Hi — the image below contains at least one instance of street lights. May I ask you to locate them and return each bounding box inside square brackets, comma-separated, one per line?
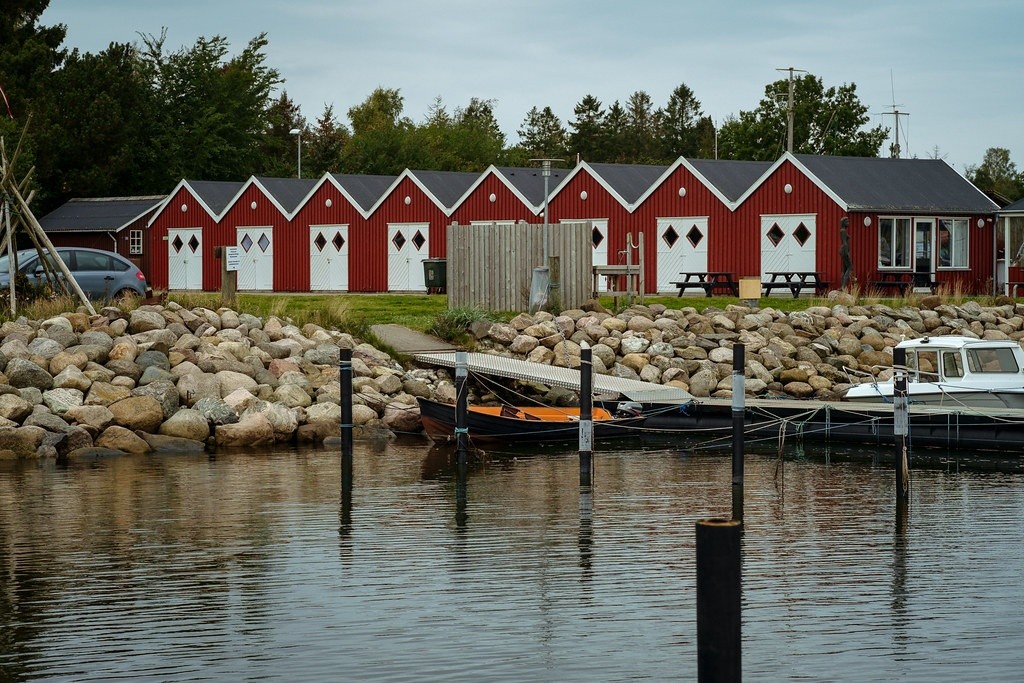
[288, 129, 302, 179]
[527, 157, 565, 268]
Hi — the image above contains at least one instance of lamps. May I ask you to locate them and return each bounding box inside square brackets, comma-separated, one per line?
[490, 193, 497, 202]
[404, 196, 411, 204]
[978, 219, 984, 228]
[580, 191, 588, 201]
[181, 204, 187, 212]
[785, 184, 793, 194]
[679, 188, 686, 197]
[864, 216, 872, 227]
[251, 201, 257, 209]
[326, 198, 332, 207]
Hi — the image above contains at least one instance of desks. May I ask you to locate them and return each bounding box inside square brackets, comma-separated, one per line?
[765, 272, 828, 299]
[875, 271, 938, 297]
[592, 264, 640, 298]
[677, 272, 739, 298]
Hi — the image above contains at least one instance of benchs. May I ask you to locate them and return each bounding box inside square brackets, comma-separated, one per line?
[670, 281, 947, 299]
[1006, 282, 1024, 299]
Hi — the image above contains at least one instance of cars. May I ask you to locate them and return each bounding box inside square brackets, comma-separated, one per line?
[0, 245, 148, 305]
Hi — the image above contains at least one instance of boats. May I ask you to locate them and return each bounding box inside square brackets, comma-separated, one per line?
[842, 334, 1024, 411]
[415, 393, 648, 448]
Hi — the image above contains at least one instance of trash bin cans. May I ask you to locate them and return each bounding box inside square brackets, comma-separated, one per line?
[422, 257, 448, 294]
